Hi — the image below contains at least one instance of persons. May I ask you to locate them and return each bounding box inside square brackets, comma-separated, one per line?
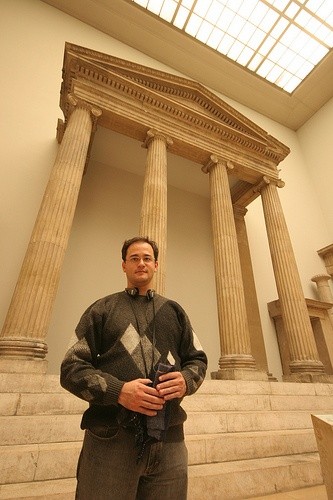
[59, 236, 208, 500]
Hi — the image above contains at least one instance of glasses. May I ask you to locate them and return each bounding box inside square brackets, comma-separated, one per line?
[124, 256, 154, 262]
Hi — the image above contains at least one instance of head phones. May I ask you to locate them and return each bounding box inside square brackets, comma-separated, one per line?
[125, 287, 155, 300]
